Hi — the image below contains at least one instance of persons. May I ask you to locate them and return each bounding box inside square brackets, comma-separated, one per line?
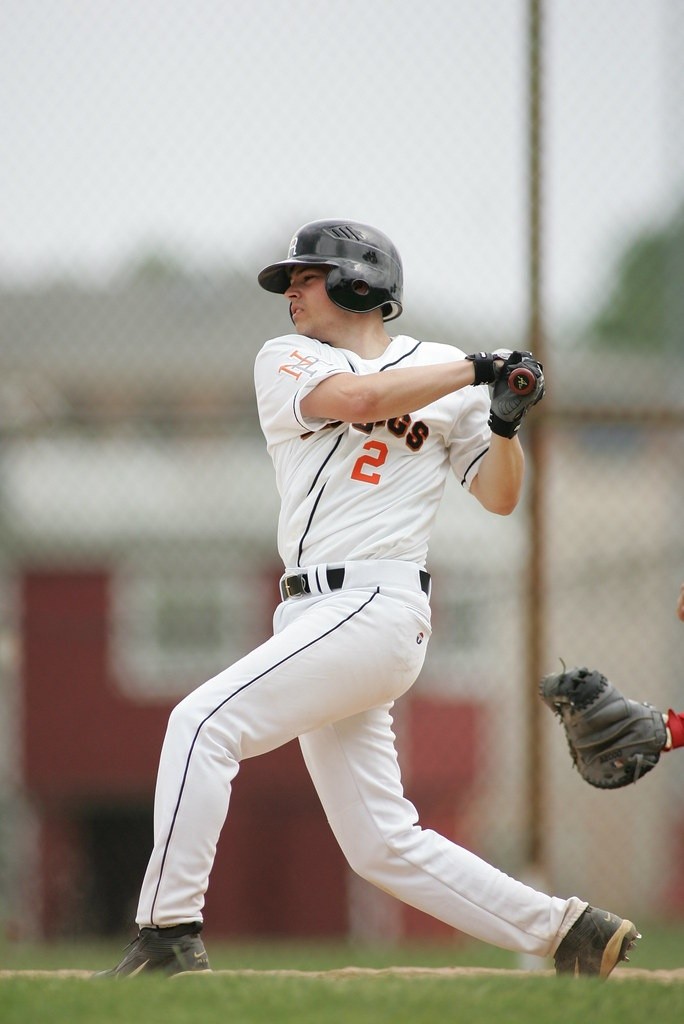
[101, 220, 642, 983]
[539, 580, 684, 789]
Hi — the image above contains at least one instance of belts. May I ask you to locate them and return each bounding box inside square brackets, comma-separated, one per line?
[280, 568, 431, 601]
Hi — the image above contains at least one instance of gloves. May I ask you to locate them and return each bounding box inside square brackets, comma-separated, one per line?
[464, 348, 543, 387]
[487, 350, 547, 440]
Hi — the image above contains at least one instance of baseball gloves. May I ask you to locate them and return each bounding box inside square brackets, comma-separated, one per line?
[537, 657, 668, 789]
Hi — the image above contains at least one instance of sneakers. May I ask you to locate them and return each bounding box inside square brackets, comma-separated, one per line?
[553, 904, 643, 984]
[89, 921, 213, 984]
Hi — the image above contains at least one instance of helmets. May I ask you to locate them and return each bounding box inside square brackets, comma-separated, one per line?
[257, 219, 404, 323]
